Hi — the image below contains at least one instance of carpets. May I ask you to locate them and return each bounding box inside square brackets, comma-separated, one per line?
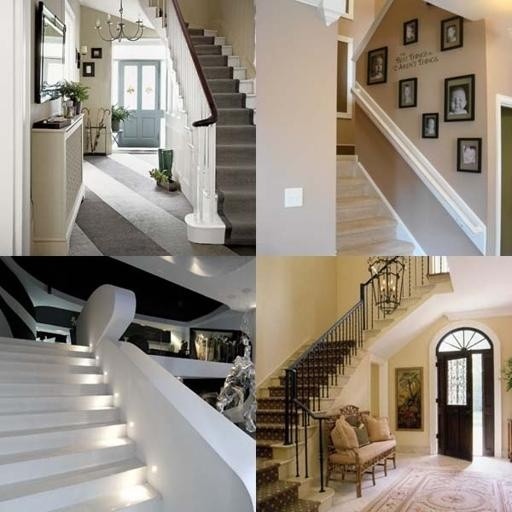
[356, 465, 512, 511]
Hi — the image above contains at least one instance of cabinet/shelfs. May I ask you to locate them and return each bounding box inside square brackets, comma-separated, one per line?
[31, 113, 85, 241]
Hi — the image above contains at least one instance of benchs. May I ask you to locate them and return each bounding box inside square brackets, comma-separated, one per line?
[322, 404, 398, 499]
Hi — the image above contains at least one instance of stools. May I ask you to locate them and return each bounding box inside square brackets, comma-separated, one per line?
[111, 129, 125, 148]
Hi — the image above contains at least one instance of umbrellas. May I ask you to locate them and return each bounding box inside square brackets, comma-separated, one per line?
[81, 105, 113, 153]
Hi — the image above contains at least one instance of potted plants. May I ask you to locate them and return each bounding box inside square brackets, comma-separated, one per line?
[111, 102, 130, 131]
[62, 79, 92, 114]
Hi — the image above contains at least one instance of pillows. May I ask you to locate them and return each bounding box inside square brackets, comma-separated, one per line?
[362, 413, 394, 442]
[328, 414, 359, 451]
[351, 422, 369, 449]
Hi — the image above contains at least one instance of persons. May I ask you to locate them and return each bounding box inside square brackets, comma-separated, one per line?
[461, 147, 475, 167]
[405, 23, 415, 41]
[445, 25, 457, 41]
[401, 84, 412, 101]
[370, 56, 383, 80]
[448, 86, 466, 116]
[422, 117, 436, 136]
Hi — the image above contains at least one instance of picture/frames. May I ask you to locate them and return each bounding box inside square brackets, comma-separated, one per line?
[402, 16, 420, 46]
[421, 112, 440, 139]
[456, 137, 482, 174]
[440, 14, 464, 52]
[444, 74, 477, 122]
[397, 76, 418, 110]
[367, 46, 388, 86]
[82, 62, 95, 77]
[91, 47, 103, 59]
[394, 366, 425, 432]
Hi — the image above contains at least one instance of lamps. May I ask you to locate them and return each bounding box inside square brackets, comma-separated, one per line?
[367, 256, 405, 316]
[75, 44, 88, 68]
[34, 1, 67, 105]
[93, 0, 145, 44]
